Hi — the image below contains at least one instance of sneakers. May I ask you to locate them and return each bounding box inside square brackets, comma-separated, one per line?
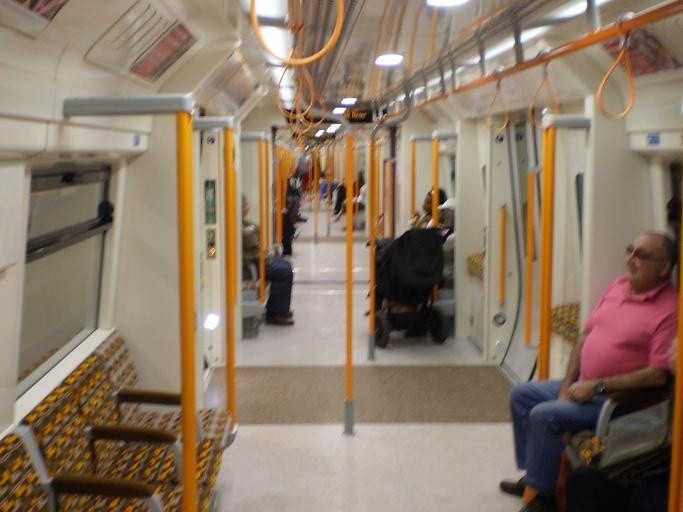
[264, 308, 295, 326]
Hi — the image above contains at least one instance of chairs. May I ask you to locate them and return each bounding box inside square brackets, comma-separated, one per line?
[23, 328, 235, 495]
[243, 248, 280, 338]
[0, 431, 166, 512]
[563, 375, 673, 512]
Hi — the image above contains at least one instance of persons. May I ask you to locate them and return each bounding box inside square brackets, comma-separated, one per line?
[498, 226, 681, 512]
[433, 202, 454, 290]
[240, 193, 298, 328]
[282, 161, 370, 259]
[366, 187, 447, 317]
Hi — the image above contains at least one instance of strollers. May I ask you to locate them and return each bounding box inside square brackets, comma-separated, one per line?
[364, 225, 455, 349]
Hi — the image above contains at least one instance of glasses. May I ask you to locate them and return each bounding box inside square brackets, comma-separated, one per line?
[625, 244, 671, 265]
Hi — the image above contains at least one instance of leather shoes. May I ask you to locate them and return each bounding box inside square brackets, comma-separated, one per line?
[499, 472, 531, 497]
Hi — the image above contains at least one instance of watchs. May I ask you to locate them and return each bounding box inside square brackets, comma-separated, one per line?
[594, 376, 611, 396]
